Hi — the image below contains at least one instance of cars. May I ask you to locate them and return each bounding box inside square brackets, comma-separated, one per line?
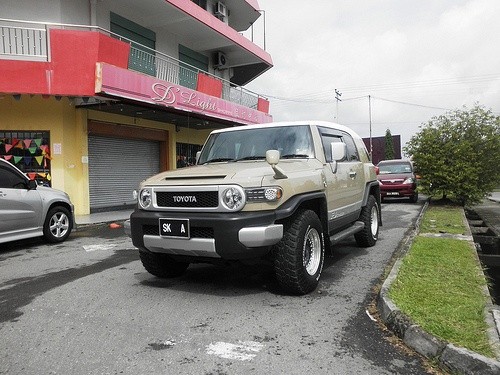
[375, 159, 421, 203]
[0, 159, 75, 245]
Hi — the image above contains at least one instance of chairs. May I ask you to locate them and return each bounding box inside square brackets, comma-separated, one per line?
[253, 141, 276, 155]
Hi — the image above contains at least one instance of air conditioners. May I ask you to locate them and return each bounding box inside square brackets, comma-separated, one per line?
[212, 51, 229, 71]
[213, 1, 227, 17]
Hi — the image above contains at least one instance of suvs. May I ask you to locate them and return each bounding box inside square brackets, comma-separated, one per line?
[129, 120, 382, 294]
